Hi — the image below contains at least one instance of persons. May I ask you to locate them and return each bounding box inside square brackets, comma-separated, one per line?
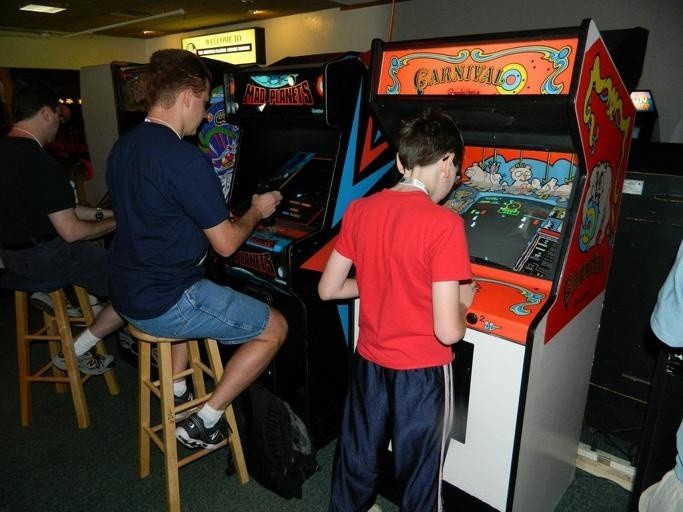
[101, 46, 293, 450]
[634, 240, 683, 512]
[1, 82, 128, 377]
[314, 111, 482, 511]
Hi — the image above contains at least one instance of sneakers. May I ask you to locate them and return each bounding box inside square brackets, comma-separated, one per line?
[28, 290, 105, 318]
[52, 348, 115, 375]
[173, 386, 195, 406]
[368, 504, 383, 512]
[174, 411, 229, 450]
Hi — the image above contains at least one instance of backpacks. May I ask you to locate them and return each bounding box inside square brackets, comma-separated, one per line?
[232, 379, 322, 501]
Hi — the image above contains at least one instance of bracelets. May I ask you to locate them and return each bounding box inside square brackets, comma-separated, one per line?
[95, 208, 103, 222]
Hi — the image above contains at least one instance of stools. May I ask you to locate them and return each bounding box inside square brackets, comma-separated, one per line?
[127, 322, 248, 512]
[2, 272, 119, 430]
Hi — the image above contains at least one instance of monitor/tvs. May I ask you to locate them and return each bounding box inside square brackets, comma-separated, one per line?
[271, 151, 335, 227]
[456, 196, 555, 272]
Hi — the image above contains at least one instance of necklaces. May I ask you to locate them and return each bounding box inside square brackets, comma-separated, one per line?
[145, 116, 181, 141]
[11, 126, 43, 150]
